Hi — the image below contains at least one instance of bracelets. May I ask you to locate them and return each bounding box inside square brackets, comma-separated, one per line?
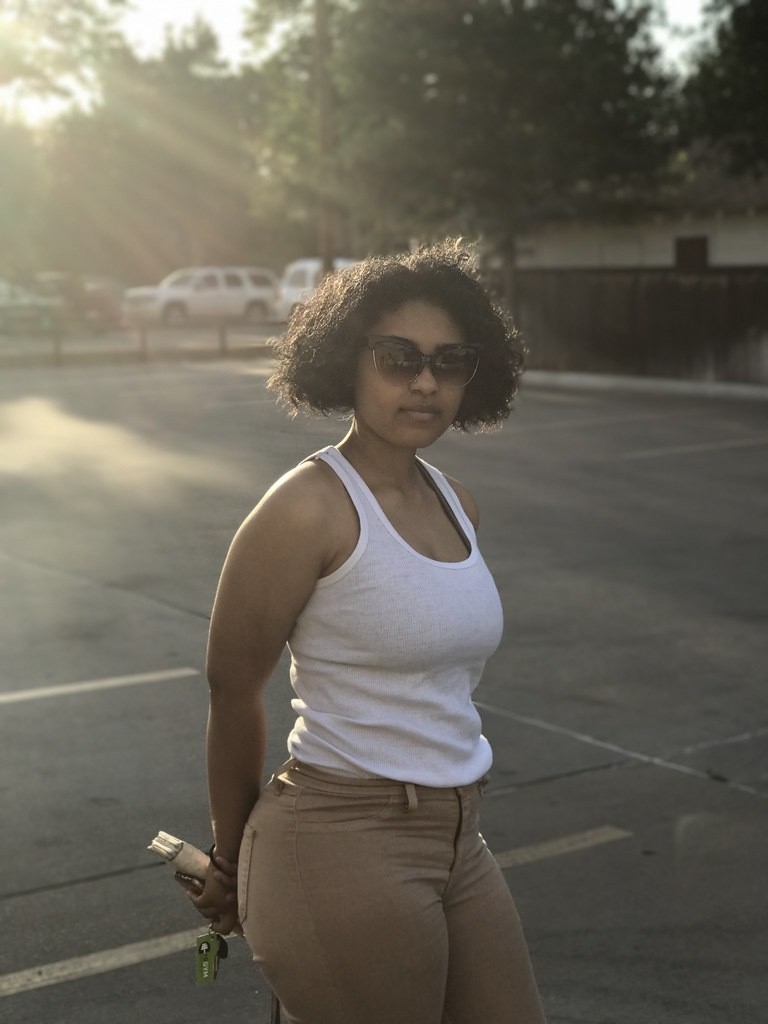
[210, 844, 238, 877]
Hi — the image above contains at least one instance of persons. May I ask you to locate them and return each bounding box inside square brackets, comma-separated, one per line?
[187, 234, 545, 1024]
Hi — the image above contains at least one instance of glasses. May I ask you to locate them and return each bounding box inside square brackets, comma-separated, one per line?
[362, 341, 484, 389]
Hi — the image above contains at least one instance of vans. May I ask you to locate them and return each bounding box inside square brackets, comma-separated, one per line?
[125, 265, 280, 328]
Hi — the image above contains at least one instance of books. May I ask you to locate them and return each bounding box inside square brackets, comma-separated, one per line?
[147, 831, 246, 939]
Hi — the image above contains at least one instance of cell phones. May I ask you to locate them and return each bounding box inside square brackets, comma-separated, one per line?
[173, 871, 205, 894]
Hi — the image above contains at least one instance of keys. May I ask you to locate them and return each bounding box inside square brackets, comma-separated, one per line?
[195, 921, 228, 985]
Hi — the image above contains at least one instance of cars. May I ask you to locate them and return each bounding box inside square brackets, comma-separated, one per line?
[266, 261, 366, 326]
